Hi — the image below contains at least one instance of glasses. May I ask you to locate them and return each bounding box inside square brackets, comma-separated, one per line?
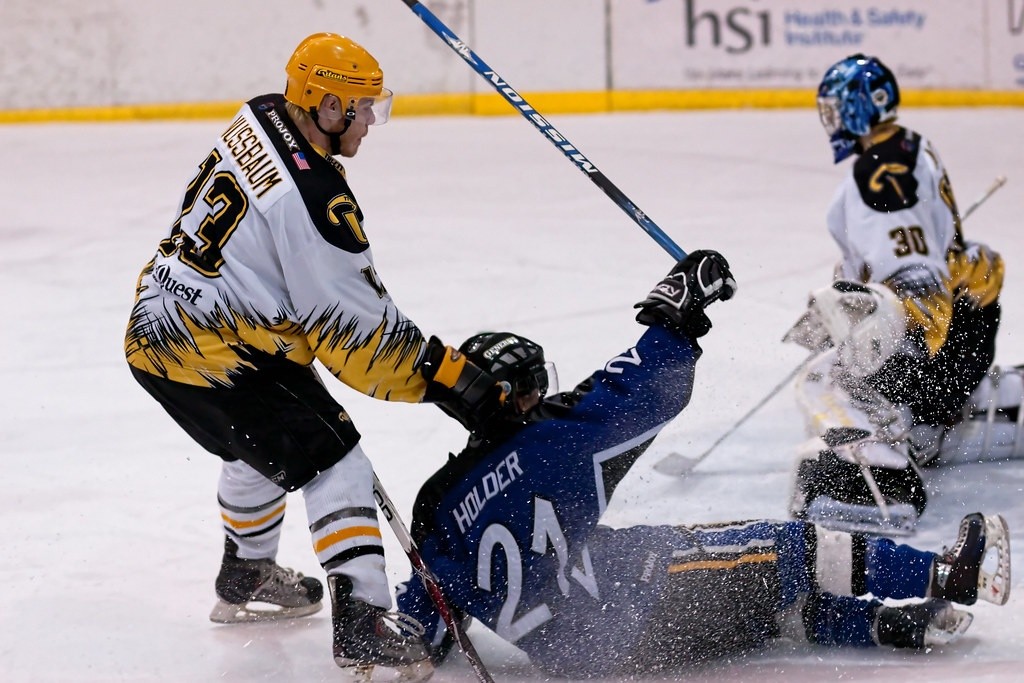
[816, 103, 841, 128]
[513, 368, 549, 396]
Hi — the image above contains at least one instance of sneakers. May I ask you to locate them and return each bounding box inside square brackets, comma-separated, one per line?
[210, 535, 325, 625]
[931, 512, 1011, 607]
[327, 574, 436, 683]
[878, 596, 974, 654]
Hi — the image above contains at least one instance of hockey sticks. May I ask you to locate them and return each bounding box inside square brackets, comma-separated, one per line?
[308, 362, 492, 683]
[402, 0, 736, 300]
[654, 175, 1005, 477]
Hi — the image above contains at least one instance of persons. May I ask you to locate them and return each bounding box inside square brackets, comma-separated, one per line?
[395, 249, 1010, 683]
[796, 53, 1024, 518]
[124, 32, 518, 683]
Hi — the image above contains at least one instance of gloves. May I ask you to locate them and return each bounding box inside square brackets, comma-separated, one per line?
[635, 249, 736, 340]
[420, 335, 508, 438]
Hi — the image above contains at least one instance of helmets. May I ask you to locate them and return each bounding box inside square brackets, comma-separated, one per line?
[816, 54, 900, 165]
[283, 32, 385, 117]
[456, 333, 546, 399]
